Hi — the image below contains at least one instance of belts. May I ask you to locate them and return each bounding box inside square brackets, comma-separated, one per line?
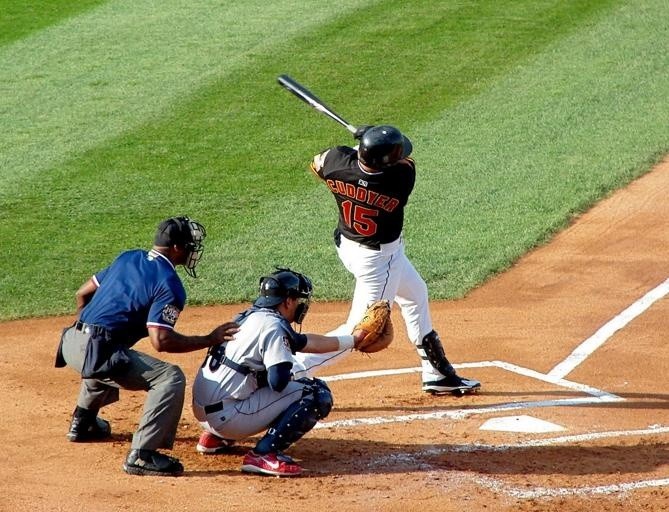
[69, 319, 130, 345]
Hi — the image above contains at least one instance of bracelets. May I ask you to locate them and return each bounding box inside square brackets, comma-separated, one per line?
[336, 334, 355, 351]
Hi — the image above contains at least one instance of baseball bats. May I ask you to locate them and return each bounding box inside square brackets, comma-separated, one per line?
[276, 74, 358, 133]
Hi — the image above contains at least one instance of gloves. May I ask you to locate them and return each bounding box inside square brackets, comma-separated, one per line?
[354, 125, 376, 140]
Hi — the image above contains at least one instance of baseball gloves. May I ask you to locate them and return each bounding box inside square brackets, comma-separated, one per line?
[351, 301, 394, 352]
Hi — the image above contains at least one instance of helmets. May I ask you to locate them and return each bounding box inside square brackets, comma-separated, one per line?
[356, 123, 415, 170]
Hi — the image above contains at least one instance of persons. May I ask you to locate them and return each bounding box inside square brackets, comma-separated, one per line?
[60, 217, 240, 477]
[285, 126, 484, 397]
[191, 266, 395, 478]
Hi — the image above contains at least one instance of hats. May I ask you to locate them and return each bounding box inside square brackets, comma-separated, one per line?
[252, 265, 308, 308]
[153, 217, 194, 248]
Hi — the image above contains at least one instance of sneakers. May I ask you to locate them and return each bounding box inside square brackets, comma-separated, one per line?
[65, 414, 114, 443]
[239, 449, 308, 476]
[421, 370, 483, 397]
[121, 447, 184, 477]
[196, 430, 238, 453]
